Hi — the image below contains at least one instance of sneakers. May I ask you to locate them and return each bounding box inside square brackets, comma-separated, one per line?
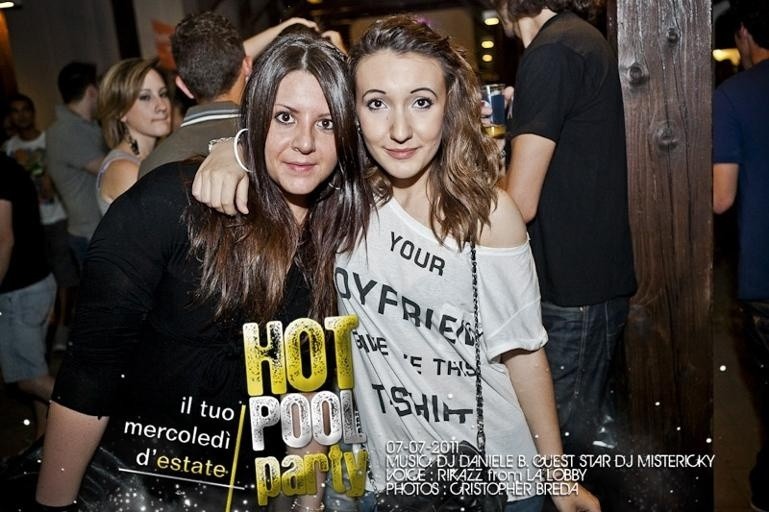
[751, 492, 769, 511]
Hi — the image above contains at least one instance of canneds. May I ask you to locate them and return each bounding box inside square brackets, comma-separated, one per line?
[480, 83, 508, 140]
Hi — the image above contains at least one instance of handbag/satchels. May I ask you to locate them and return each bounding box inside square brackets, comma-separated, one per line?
[375, 440, 508, 512]
[2, 433, 154, 511]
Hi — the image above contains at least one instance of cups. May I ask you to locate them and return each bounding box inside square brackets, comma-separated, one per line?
[480, 83, 509, 139]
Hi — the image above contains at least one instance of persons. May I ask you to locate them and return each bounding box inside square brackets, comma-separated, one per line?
[45, 62, 106, 272]
[189, 11, 605, 511]
[1, 148, 57, 469]
[94, 9, 360, 251]
[708, 11, 768, 511]
[32, 26, 370, 511]
[1, 92, 82, 357]
[487, 1, 630, 461]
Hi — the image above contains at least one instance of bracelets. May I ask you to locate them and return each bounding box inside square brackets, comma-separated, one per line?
[34, 499, 79, 511]
[206, 137, 236, 152]
[539, 455, 570, 476]
